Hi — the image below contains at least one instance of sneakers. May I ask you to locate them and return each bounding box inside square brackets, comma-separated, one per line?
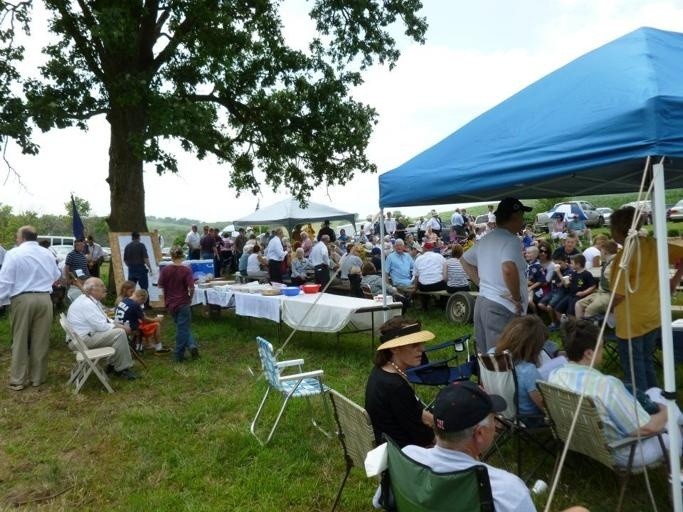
[6, 382, 41, 391]
[116, 344, 200, 381]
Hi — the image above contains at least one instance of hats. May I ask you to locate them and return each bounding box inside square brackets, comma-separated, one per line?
[170, 245, 183, 257]
[376, 322, 436, 351]
[497, 197, 532, 215]
[434, 380, 507, 433]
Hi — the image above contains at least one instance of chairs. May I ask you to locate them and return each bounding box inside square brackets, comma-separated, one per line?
[533, 374, 672, 509]
[475, 348, 538, 476]
[59, 312, 115, 395]
[250, 336, 334, 449]
[325, 389, 391, 510]
[380, 428, 501, 512]
[392, 331, 476, 416]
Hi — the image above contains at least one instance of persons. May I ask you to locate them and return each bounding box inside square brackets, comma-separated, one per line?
[459, 196, 533, 354]
[484, 314, 566, 422]
[188, 205, 623, 319]
[545, 317, 682, 475]
[1, 225, 200, 392]
[607, 206, 682, 392]
[370, 380, 589, 512]
[365, 314, 440, 455]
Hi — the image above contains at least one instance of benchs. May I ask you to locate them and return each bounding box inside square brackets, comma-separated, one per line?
[408, 255, 683, 344]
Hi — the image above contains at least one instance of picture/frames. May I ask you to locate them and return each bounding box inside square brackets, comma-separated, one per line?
[106, 230, 172, 312]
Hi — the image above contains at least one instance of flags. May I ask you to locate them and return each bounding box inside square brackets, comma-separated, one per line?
[69, 196, 85, 241]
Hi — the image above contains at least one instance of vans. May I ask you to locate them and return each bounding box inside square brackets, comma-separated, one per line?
[34, 235, 87, 260]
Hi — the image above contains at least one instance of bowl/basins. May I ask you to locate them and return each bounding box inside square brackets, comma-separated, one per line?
[282, 286, 300, 295]
[302, 283, 322, 294]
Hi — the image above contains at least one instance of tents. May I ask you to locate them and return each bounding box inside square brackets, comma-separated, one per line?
[379, 25, 682, 511]
[233, 195, 358, 242]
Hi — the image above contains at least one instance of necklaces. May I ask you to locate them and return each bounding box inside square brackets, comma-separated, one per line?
[386, 360, 409, 377]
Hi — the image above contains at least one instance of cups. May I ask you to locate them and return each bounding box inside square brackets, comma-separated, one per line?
[562, 276, 570, 286]
[532, 479, 547, 495]
[156, 315, 163, 323]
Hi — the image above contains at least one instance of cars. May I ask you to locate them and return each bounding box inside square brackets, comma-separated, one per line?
[160, 246, 188, 261]
[401, 197, 682, 241]
[101, 246, 111, 262]
